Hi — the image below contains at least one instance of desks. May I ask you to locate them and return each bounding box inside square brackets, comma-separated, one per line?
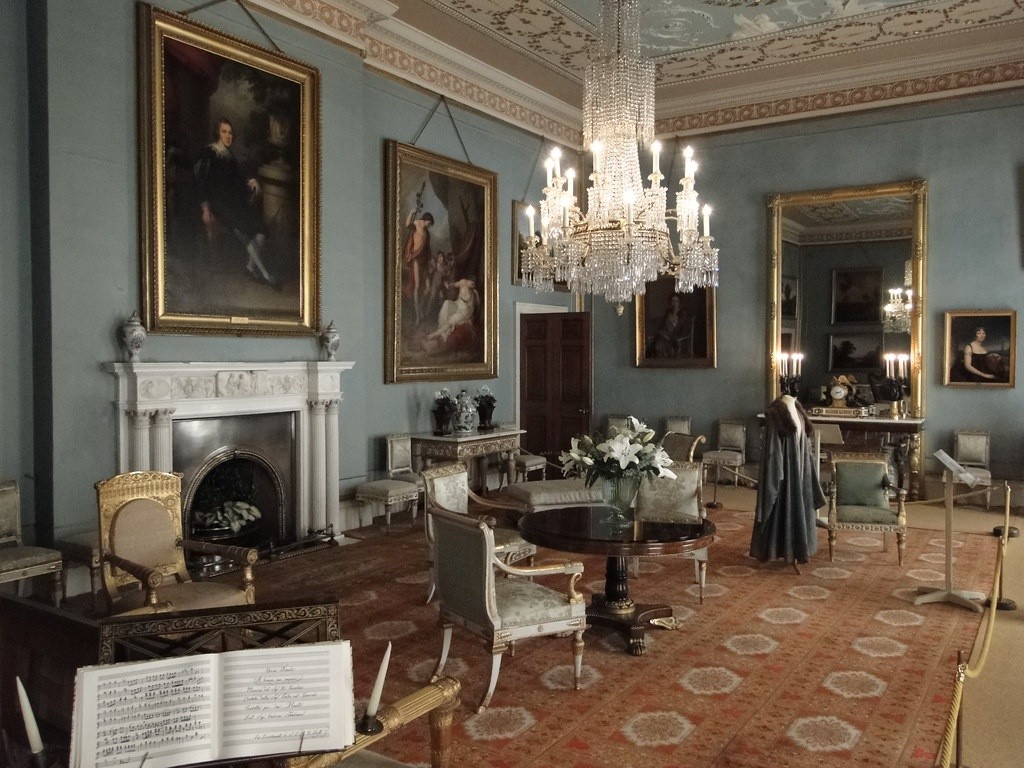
[759, 413, 925, 502]
[411, 427, 527, 495]
[518, 506, 716, 656]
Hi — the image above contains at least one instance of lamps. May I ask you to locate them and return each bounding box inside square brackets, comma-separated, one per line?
[884, 260, 912, 334]
[521, 0, 720, 316]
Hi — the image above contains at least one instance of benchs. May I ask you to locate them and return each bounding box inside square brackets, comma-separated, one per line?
[508, 477, 612, 506]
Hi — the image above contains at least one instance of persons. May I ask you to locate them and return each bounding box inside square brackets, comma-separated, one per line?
[750, 394, 826, 563]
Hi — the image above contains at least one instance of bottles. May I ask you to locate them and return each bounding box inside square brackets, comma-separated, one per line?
[319, 319, 342, 361]
[119, 309, 147, 364]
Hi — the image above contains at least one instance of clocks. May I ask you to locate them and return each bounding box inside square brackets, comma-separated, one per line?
[831, 384, 848, 407]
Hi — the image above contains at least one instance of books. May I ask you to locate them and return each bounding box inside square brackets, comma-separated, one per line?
[69, 641, 355, 768]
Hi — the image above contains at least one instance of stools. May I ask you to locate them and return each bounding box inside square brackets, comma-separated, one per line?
[356, 479, 418, 536]
[499, 455, 547, 492]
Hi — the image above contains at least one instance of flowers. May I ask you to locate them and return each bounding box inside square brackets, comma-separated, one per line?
[434, 386, 457, 409]
[558, 415, 678, 488]
[474, 385, 497, 406]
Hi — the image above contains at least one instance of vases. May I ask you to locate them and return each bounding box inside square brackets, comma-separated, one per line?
[598, 469, 641, 528]
[432, 410, 455, 436]
[477, 406, 497, 429]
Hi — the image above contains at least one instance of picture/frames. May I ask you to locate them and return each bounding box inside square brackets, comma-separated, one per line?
[136, 0, 322, 339]
[942, 309, 1017, 388]
[782, 275, 799, 320]
[511, 198, 572, 292]
[780, 328, 797, 375]
[828, 331, 885, 373]
[634, 271, 718, 371]
[831, 265, 885, 325]
[384, 139, 500, 385]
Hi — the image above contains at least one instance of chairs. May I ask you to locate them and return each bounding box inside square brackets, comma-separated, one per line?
[0, 480, 65, 607]
[418, 463, 537, 604]
[427, 508, 586, 715]
[942, 427, 992, 510]
[702, 419, 746, 488]
[94, 471, 260, 647]
[659, 431, 707, 462]
[664, 417, 691, 434]
[634, 461, 707, 584]
[385, 432, 428, 511]
[828, 452, 908, 565]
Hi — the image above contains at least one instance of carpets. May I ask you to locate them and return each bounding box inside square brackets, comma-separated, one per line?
[1, 484, 1002, 768]
[0, 591, 218, 768]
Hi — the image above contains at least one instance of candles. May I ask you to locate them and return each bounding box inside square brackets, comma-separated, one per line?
[15, 676, 43, 754]
[791, 353, 797, 377]
[766, 178, 928, 422]
[883, 354, 909, 381]
[367, 640, 392, 715]
[781, 353, 803, 376]
